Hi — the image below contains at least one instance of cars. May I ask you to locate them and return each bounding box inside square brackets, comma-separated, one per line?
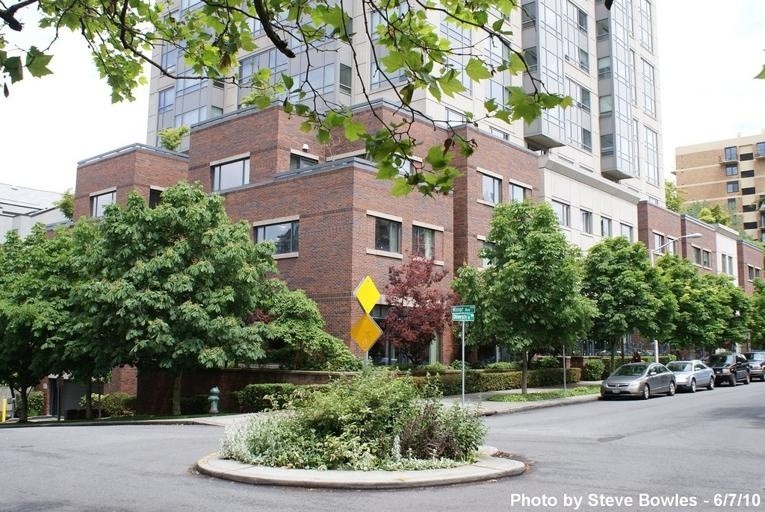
[588, 343, 669, 357]
[665, 359, 716, 393]
[598, 361, 678, 401]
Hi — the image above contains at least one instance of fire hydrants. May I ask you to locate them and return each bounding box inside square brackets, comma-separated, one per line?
[207, 386, 222, 414]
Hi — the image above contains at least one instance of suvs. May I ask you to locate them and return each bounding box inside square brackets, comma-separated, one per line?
[743, 350, 765, 383]
[705, 351, 753, 387]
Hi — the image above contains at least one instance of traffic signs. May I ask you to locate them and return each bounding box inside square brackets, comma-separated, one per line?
[452, 312, 475, 322]
[450, 304, 476, 314]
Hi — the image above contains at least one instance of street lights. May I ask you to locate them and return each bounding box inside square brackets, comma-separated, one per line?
[647, 231, 708, 369]
